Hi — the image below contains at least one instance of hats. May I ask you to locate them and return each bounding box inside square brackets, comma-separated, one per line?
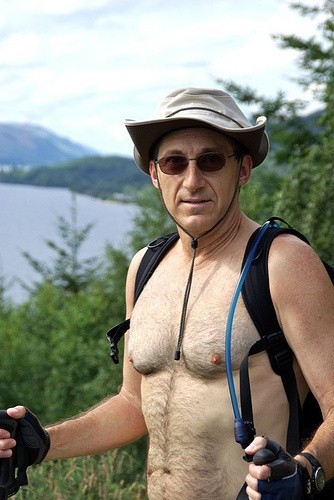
[123, 87, 269, 176]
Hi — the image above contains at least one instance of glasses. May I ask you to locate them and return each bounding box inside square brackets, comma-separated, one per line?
[155, 150, 240, 175]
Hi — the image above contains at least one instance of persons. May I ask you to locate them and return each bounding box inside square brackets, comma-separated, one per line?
[0, 90, 334, 499]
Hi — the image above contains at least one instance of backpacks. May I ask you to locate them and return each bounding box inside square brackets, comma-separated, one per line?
[108, 216, 334, 499]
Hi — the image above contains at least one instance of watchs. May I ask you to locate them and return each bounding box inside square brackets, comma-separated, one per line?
[299, 451, 327, 498]
